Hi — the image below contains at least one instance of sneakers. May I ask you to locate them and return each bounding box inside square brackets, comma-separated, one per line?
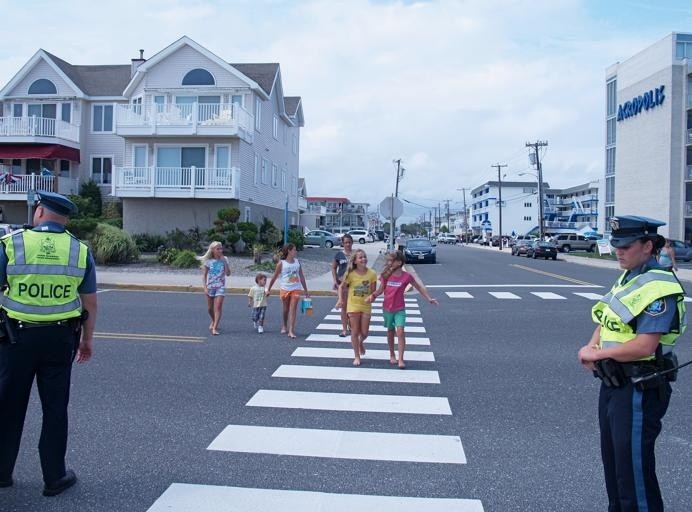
[339, 330, 348, 337]
[258, 326, 263, 333]
[348, 326, 351, 335]
[254, 321, 258, 328]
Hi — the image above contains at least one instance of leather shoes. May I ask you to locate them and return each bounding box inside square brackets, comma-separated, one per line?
[0, 480, 13, 488]
[43, 471, 76, 497]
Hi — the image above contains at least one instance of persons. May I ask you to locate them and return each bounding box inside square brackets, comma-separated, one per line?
[194, 241, 231, 335]
[0, 190, 97, 497]
[335, 249, 377, 365]
[656, 239, 678, 271]
[578, 213, 692, 512]
[332, 234, 357, 337]
[248, 273, 269, 333]
[265, 243, 308, 339]
[364, 250, 439, 368]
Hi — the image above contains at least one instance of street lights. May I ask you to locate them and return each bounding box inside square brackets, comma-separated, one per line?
[456, 186, 470, 244]
[518, 171, 545, 241]
[499, 171, 508, 239]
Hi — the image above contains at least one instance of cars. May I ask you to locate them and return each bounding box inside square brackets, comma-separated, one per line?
[437, 230, 457, 246]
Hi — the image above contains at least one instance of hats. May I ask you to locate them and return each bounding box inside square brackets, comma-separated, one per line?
[35, 190, 78, 217]
[605, 215, 666, 248]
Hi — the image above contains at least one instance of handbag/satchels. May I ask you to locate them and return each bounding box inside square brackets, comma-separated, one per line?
[302, 295, 312, 314]
